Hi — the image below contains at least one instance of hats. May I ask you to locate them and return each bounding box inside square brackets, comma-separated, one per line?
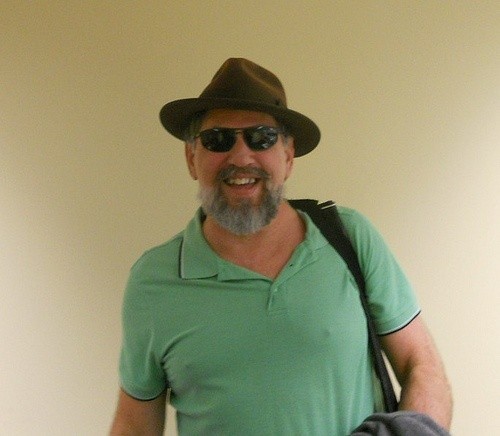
[160, 57, 321, 158]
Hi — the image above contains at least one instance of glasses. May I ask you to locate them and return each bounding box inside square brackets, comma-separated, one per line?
[194, 124, 288, 153]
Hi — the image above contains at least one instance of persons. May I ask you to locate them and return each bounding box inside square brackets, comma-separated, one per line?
[110, 57, 454, 436]
